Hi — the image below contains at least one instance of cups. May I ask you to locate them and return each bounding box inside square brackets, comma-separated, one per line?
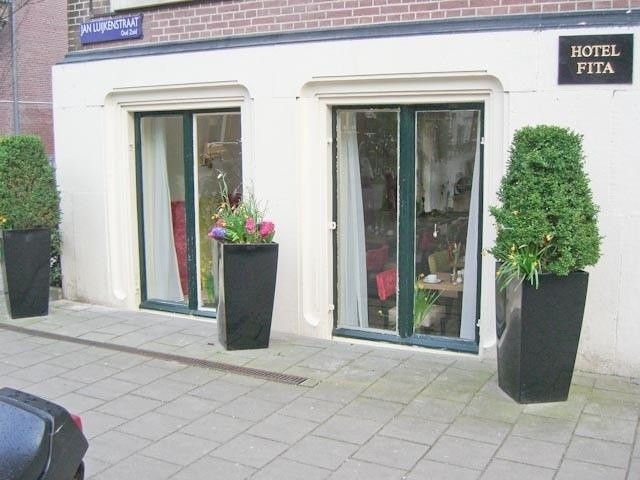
[425, 275, 438, 282]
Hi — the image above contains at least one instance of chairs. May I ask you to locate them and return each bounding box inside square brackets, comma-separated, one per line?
[364, 212, 466, 340]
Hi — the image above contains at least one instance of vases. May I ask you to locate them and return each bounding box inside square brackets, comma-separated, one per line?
[213, 241, 279, 352]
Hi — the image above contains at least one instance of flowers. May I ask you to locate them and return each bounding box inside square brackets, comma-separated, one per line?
[209, 169, 276, 245]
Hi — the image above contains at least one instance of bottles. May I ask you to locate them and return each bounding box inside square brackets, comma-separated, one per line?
[455, 270, 463, 286]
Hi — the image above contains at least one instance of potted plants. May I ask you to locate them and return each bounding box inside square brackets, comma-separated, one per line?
[486, 124, 605, 404]
[0, 134, 61, 319]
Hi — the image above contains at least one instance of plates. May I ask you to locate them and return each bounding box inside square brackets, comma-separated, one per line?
[423, 279, 441, 284]
